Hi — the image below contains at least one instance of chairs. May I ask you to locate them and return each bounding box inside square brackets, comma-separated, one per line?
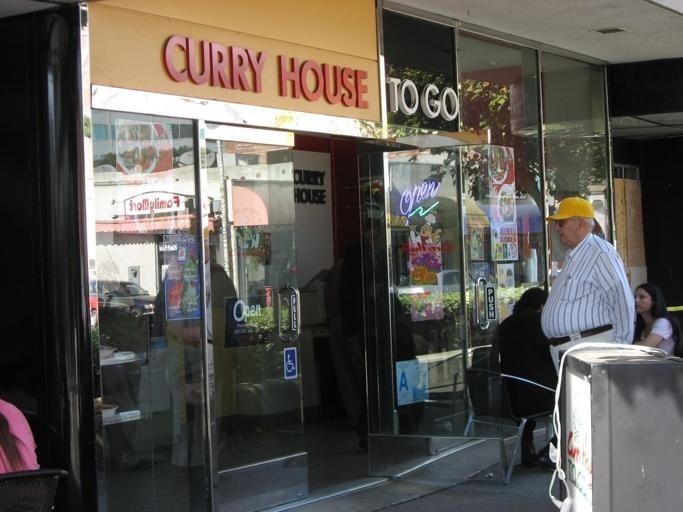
[0, 469, 70, 512]
[456, 362, 558, 486]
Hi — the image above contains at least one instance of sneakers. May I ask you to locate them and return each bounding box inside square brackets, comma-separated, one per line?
[520, 444, 539, 468]
[536, 442, 556, 472]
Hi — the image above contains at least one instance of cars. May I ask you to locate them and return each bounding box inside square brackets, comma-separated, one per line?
[394, 266, 489, 295]
[90, 276, 158, 336]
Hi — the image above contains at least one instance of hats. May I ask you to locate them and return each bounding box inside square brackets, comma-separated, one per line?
[544, 196, 596, 224]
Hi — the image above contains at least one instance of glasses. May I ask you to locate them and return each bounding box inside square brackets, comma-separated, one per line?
[553, 220, 578, 227]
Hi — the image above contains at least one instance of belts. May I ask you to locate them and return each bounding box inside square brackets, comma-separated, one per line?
[545, 322, 614, 349]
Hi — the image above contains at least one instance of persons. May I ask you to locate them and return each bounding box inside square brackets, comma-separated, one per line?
[633, 283, 683, 358]
[0, 397, 54, 512]
[541, 197, 634, 374]
[492, 288, 559, 471]
[152, 236, 246, 482]
[98, 267, 157, 472]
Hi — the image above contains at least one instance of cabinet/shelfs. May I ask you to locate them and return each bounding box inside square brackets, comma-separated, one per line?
[98, 306, 156, 429]
